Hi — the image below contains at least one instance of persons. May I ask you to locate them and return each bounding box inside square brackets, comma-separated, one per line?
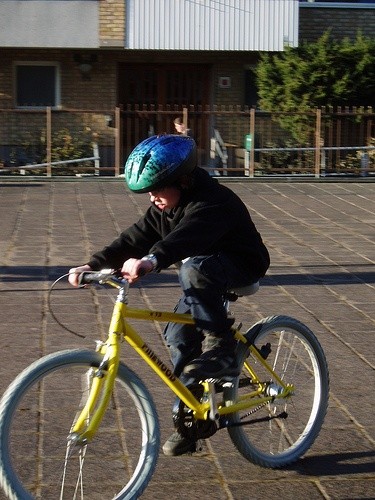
[174, 117, 192, 135]
[69, 134, 269, 456]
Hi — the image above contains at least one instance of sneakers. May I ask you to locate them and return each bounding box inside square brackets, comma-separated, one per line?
[161, 427, 195, 457]
[183, 328, 240, 375]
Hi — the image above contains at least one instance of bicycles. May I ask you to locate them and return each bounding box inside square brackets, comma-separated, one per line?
[0, 265, 330, 500]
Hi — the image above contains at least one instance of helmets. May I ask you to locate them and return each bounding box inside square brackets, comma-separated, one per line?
[124, 133, 197, 194]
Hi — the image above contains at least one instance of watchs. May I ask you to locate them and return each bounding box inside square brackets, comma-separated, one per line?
[142, 254, 158, 271]
